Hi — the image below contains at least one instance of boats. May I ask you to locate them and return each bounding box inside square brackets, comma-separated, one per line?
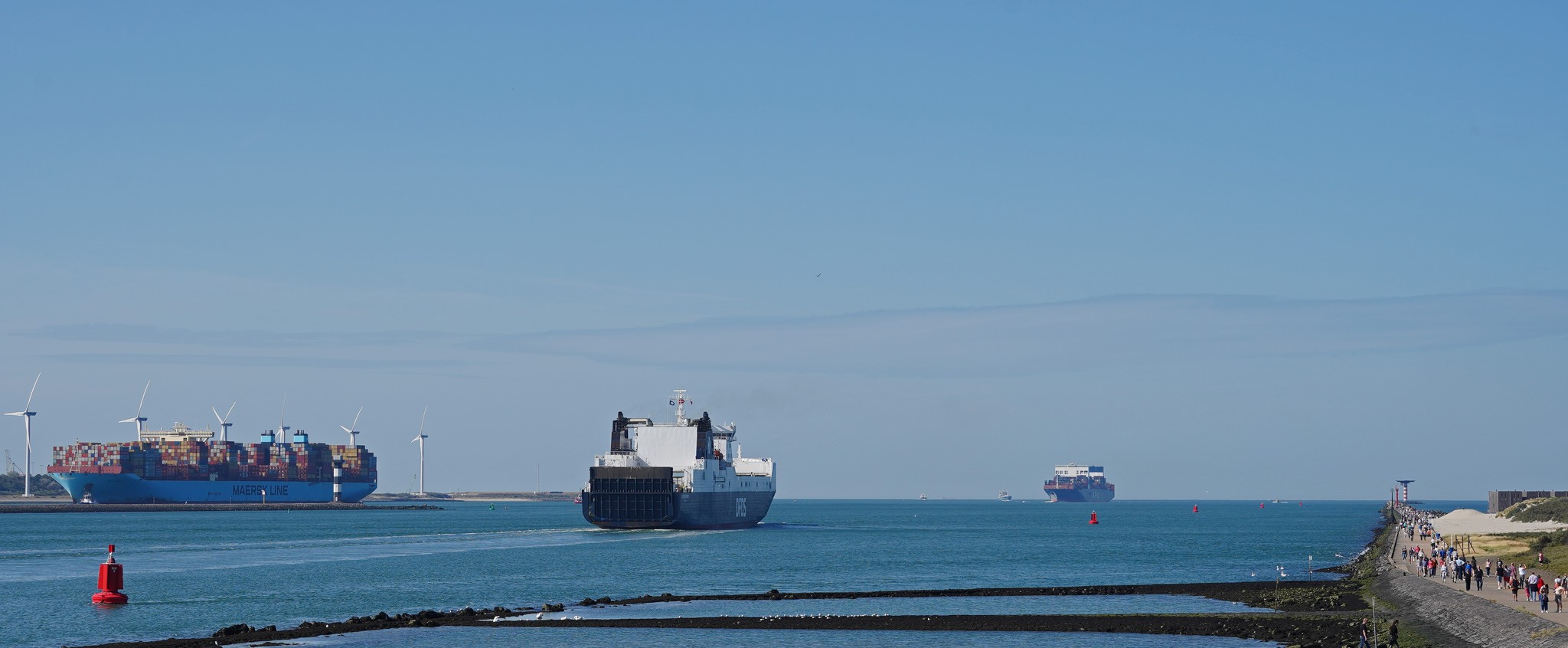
[1280, 501, 1289, 503]
[1044, 460, 1115, 502]
[920, 493, 927, 500]
[80, 491, 92, 504]
[1276, 498, 1280, 502]
[574, 487, 582, 503]
[582, 386, 777, 530]
[998, 488, 1012, 501]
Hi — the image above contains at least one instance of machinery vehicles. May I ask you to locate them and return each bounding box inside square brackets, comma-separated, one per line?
[406, 474, 416, 494]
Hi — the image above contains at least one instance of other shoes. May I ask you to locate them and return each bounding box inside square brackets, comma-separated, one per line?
[1403, 559, 1406, 562]
[1522, 588, 1524, 589]
[1546, 611, 1549, 613]
[1442, 581, 1445, 583]
[1566, 594, 1568, 598]
[1417, 574, 1421, 577]
[1477, 587, 1482, 591]
[1452, 580, 1456, 583]
[1432, 575, 1436, 577]
[1533, 600, 1536, 602]
[1501, 586, 1502, 589]
[1541, 611, 1544, 613]
[1555, 610, 1559, 612]
[1503, 587, 1506, 589]
[1508, 586, 1510, 589]
[1513, 600, 1517, 602]
[1410, 562, 1412, 564]
[1447, 564, 1450, 566]
[1559, 610, 1562, 612]
[1564, 596, 1566, 598]
[1530, 600, 1533, 601]
[1498, 587, 1500, 589]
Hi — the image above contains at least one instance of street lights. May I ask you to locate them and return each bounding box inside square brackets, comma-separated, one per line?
[39, 465, 43, 475]
[31, 462, 35, 476]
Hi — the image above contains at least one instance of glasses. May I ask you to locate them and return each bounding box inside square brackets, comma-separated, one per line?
[1556, 584, 1558, 585]
[1397, 622, 1399, 624]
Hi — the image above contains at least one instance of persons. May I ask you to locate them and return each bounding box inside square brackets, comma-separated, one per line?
[1430, 537, 1435, 550]
[1539, 584, 1549, 613]
[1395, 506, 1443, 545]
[1554, 581, 1565, 612]
[1538, 551, 1543, 568]
[1389, 620, 1400, 648]
[1359, 618, 1371, 648]
[1457, 506, 1465, 509]
[1401, 545, 1568, 602]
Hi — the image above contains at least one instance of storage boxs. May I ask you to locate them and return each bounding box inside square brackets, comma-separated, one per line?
[53, 441, 377, 482]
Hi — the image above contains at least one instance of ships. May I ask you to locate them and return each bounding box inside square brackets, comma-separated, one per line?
[47, 421, 377, 503]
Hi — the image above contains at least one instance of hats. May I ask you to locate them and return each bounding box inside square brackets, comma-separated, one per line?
[1519, 564, 1522, 566]
[1429, 558, 1432, 560]
[1453, 560, 1455, 561]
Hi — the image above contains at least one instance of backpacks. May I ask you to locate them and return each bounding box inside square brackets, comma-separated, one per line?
[1512, 579, 1518, 588]
